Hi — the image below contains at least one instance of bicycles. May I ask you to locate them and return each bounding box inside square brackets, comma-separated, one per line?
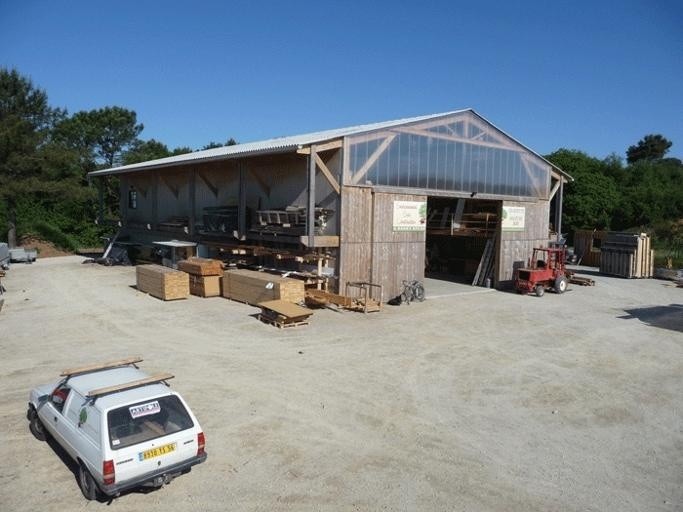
[402, 279, 424, 305]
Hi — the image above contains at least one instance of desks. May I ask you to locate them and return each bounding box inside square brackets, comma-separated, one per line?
[257, 299, 314, 329]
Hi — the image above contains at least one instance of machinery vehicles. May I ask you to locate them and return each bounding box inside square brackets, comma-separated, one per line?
[513, 244, 574, 297]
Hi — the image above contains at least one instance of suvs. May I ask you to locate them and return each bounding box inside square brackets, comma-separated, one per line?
[28, 355, 208, 502]
